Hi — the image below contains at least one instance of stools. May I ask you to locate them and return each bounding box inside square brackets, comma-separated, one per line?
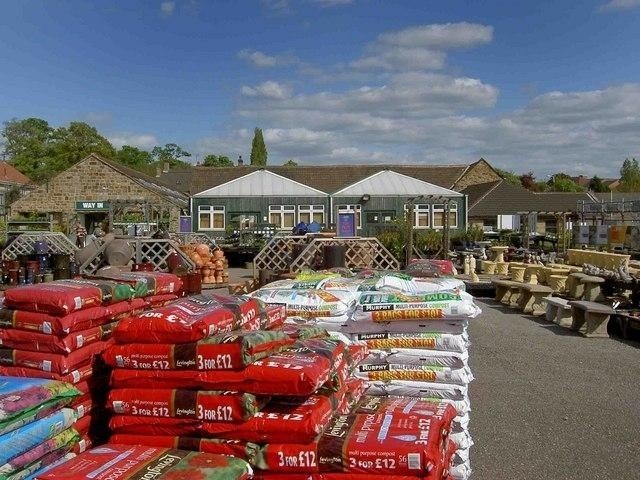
[490, 277, 618, 340]
